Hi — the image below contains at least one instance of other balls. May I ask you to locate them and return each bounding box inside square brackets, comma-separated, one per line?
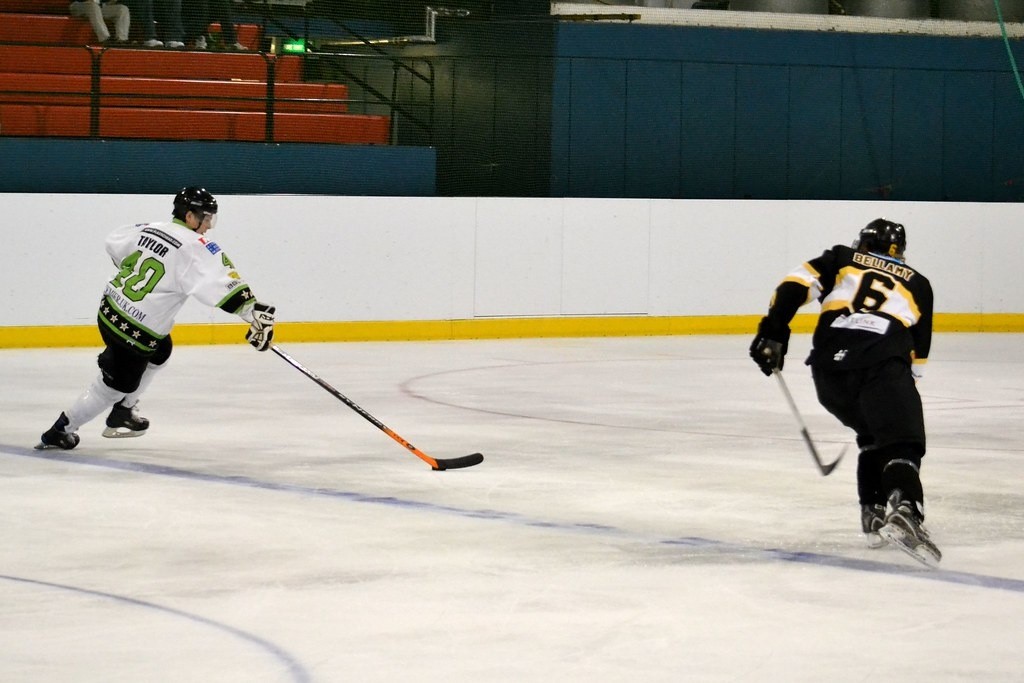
[432, 466, 446, 471]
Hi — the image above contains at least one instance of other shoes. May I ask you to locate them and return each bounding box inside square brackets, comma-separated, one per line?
[232, 42, 248, 50]
[99, 37, 140, 49]
[142, 37, 186, 51]
[195, 35, 208, 50]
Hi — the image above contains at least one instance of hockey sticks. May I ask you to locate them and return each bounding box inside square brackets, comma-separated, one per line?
[765, 347, 848, 476]
[269, 339, 484, 470]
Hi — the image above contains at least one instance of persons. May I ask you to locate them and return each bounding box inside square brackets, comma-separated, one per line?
[69, 0, 249, 52]
[748, 218, 942, 567]
[34, 187, 275, 451]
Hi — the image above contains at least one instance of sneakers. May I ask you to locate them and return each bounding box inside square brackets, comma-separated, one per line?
[862, 501, 943, 562]
[42, 413, 80, 448]
[105, 397, 150, 432]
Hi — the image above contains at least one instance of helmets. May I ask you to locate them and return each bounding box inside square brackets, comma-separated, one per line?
[174, 185, 218, 213]
[860, 217, 908, 257]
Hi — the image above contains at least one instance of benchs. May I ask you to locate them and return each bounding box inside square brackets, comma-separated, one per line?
[1, 0, 391, 145]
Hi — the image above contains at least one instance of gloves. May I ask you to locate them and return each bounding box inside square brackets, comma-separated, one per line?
[749, 316, 791, 376]
[245, 303, 277, 352]
